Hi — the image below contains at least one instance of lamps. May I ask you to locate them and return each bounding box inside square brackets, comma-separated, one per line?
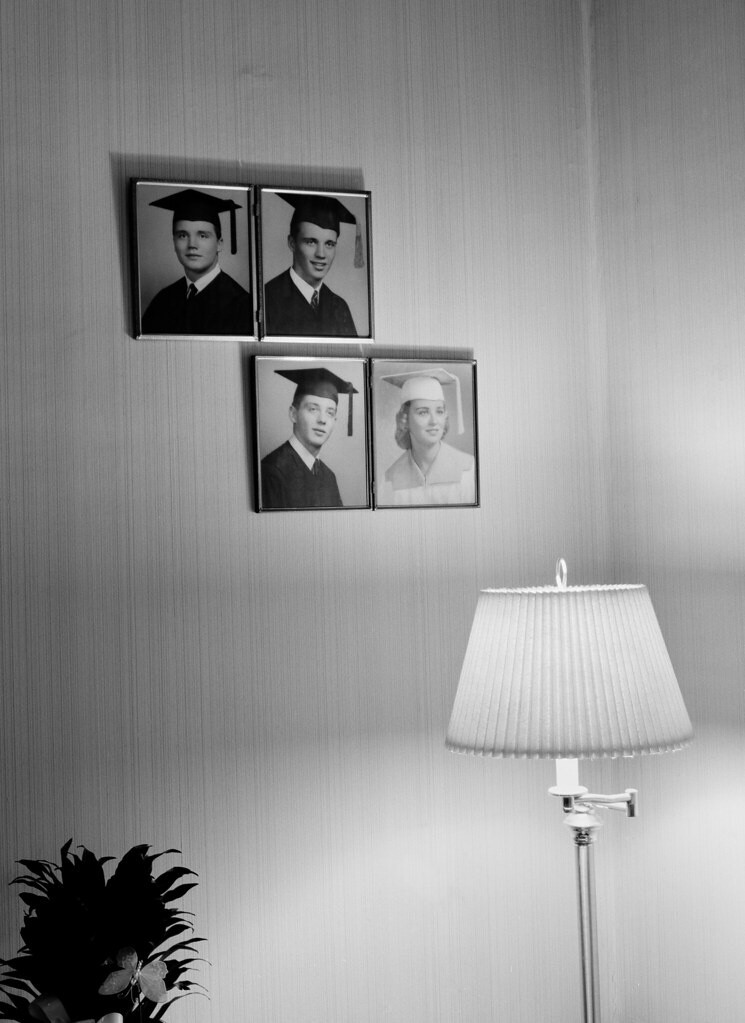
[443, 557, 696, 1023]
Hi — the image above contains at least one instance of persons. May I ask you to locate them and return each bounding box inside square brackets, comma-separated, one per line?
[260, 367, 358, 507]
[141, 189, 254, 336]
[378, 368, 476, 505]
[264, 192, 364, 336]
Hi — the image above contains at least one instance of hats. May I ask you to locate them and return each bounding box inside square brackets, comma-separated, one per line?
[274, 193, 366, 270]
[274, 367, 359, 436]
[380, 369, 466, 435]
[149, 189, 242, 255]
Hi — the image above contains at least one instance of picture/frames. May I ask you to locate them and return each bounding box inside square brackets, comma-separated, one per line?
[127, 176, 259, 342]
[364, 359, 479, 509]
[248, 354, 370, 512]
[254, 184, 376, 345]
